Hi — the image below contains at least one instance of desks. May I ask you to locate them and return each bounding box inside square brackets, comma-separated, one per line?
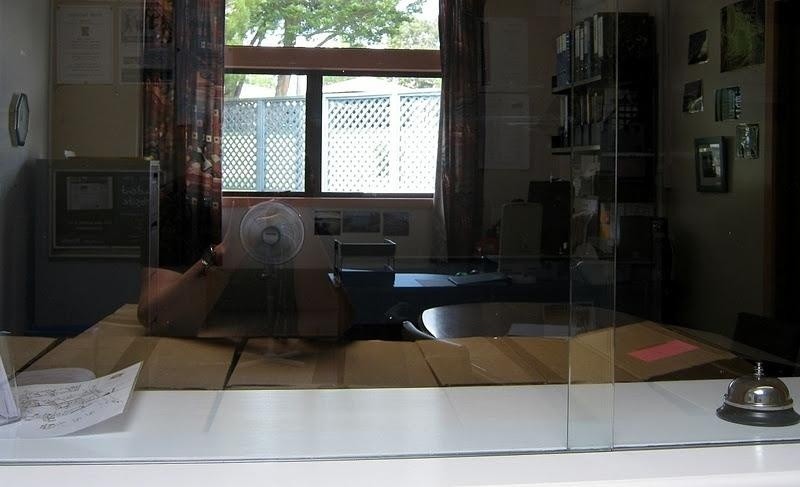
[328, 266, 574, 336]
[422, 299, 698, 382]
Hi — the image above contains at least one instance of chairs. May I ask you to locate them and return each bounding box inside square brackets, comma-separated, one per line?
[394, 318, 471, 388]
[730, 310, 800, 378]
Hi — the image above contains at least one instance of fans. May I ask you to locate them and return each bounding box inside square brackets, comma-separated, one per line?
[238, 200, 305, 336]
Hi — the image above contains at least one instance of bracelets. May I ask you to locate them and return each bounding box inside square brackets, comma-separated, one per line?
[197, 237, 238, 274]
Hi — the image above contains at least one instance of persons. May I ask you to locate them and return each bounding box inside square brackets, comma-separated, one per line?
[135, 160, 357, 343]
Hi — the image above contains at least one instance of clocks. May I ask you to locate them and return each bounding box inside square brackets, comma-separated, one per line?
[9, 91, 30, 147]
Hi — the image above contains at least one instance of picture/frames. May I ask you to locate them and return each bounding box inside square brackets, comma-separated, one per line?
[694, 136, 730, 194]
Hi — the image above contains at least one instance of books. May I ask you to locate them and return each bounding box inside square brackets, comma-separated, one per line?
[499, 318, 739, 384]
[341, 334, 547, 385]
[547, 12, 606, 145]
[110, 331, 339, 388]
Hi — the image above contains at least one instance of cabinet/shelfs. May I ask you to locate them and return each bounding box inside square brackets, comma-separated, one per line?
[550, 10, 654, 304]
[331, 235, 397, 289]
[28, 156, 159, 336]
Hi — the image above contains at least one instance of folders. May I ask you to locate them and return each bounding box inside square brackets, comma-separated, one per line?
[555, 12, 616, 87]
